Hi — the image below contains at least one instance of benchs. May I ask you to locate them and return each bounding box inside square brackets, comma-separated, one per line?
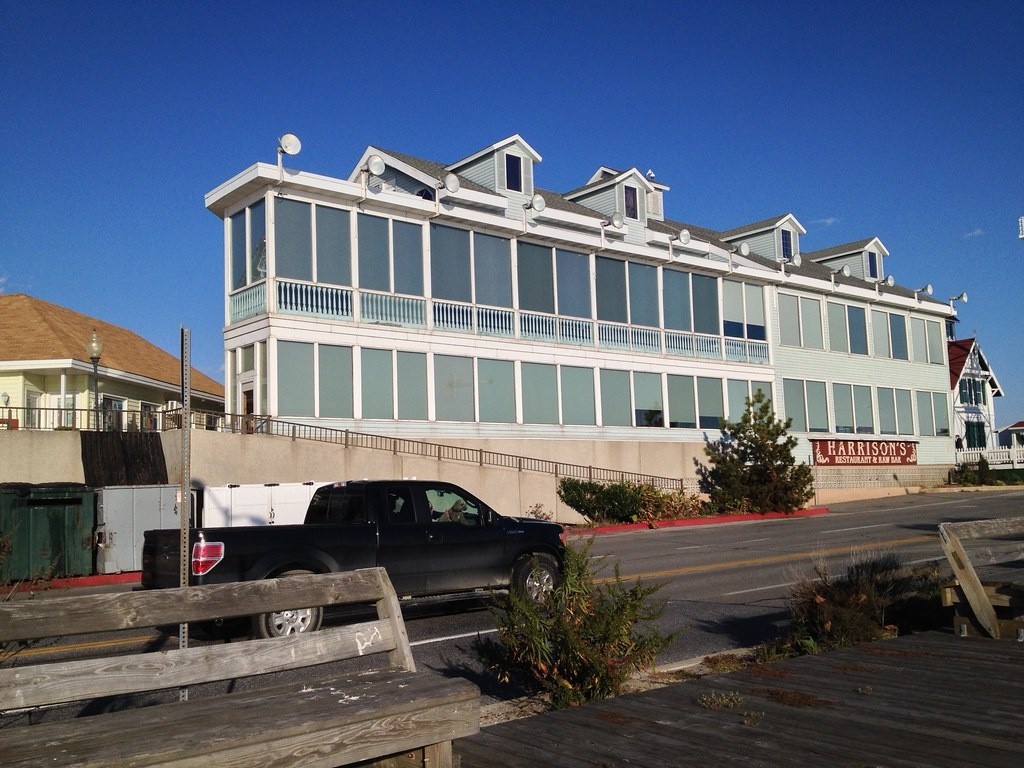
[0, 567, 482, 768]
[937, 516, 1024, 639]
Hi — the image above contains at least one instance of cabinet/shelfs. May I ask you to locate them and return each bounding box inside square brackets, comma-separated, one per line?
[92, 483, 183, 574]
[200, 480, 335, 529]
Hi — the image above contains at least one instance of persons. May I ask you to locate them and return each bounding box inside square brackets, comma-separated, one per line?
[429, 501, 464, 522]
[390, 495, 400, 511]
[955, 434, 964, 452]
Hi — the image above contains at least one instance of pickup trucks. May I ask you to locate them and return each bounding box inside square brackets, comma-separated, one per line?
[140, 479, 568, 639]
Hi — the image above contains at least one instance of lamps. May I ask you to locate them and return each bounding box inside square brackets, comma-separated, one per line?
[774, 253, 968, 318]
[276, 133, 302, 187]
[666, 228, 691, 263]
[2, 392, 10, 406]
[725, 241, 750, 277]
[427, 174, 460, 217]
[598, 212, 625, 253]
[356, 155, 386, 202]
[515, 194, 546, 236]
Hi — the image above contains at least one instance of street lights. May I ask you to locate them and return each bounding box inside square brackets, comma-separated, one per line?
[86, 327, 105, 431]
